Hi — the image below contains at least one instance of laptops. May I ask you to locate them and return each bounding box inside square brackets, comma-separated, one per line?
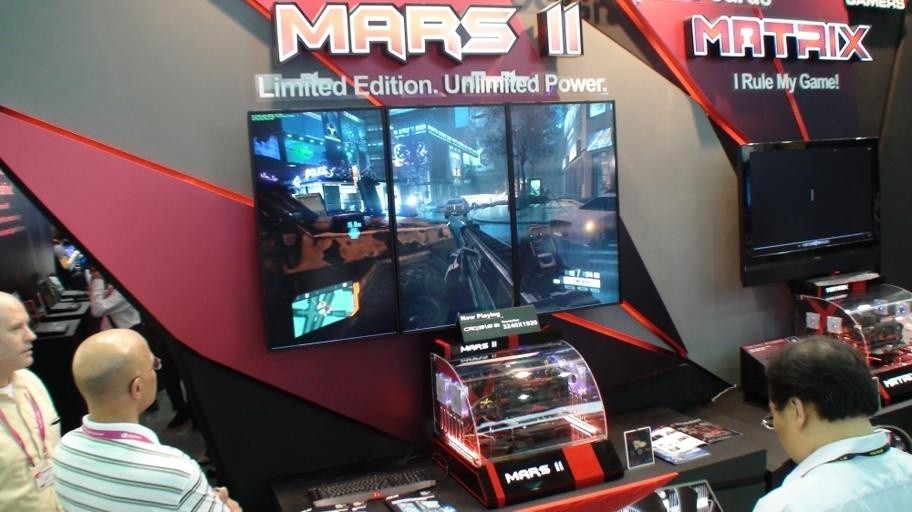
[37, 279, 80, 313]
[47, 273, 90, 298]
[12, 292, 69, 335]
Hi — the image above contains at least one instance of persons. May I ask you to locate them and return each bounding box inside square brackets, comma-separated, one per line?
[0, 290, 66, 512]
[161, 348, 201, 431]
[750, 336, 912, 512]
[53, 229, 81, 280]
[89, 268, 153, 339]
[51, 328, 243, 512]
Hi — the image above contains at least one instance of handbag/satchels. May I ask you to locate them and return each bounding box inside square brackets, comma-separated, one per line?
[129, 320, 151, 337]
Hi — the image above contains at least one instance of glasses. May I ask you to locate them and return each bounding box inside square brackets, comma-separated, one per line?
[141, 357, 162, 378]
[762, 408, 787, 430]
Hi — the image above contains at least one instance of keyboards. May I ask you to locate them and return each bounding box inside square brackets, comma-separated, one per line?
[308, 466, 436, 511]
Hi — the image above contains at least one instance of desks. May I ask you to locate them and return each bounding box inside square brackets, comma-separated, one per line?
[46, 303, 92, 318]
[268, 406, 767, 512]
[30, 319, 80, 358]
[61, 297, 91, 301]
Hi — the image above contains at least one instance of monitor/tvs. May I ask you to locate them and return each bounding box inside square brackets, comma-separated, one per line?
[738, 136, 880, 287]
[386, 103, 518, 335]
[507, 100, 623, 315]
[248, 107, 401, 352]
